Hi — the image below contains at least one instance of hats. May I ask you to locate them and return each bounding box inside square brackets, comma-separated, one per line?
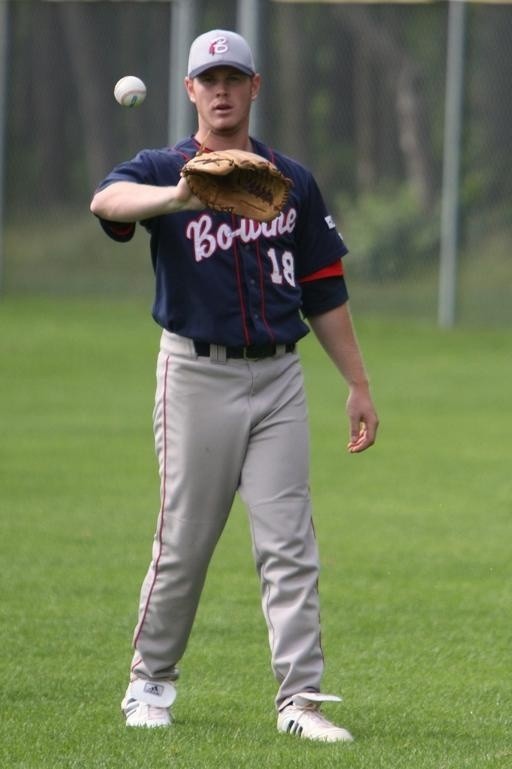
[187, 28, 257, 80]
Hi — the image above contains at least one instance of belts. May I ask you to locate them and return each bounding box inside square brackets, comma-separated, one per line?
[193, 339, 296, 358]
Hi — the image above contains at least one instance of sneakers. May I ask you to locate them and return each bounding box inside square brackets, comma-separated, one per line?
[277, 693, 353, 742]
[121, 679, 178, 727]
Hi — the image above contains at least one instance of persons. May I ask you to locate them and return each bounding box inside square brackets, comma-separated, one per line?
[85, 25, 382, 745]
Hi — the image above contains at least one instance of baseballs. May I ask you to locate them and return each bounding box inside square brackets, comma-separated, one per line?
[113, 75, 147, 110]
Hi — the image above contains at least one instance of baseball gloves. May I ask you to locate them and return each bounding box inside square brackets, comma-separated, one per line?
[186, 148, 293, 223]
